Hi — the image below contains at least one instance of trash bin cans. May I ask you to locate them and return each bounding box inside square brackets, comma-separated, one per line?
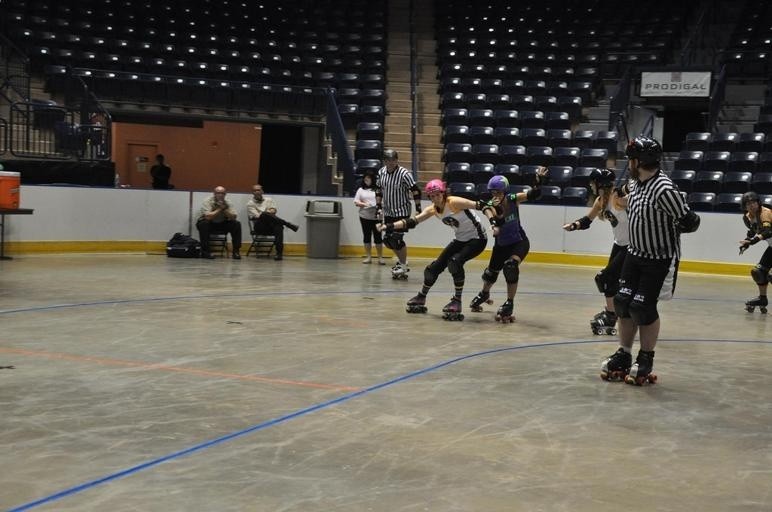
[304, 200, 344, 258]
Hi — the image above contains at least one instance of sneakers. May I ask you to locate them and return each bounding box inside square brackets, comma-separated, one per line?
[231, 250, 241, 259]
[274, 254, 283, 261]
[378, 256, 386, 264]
[291, 224, 300, 232]
[362, 256, 373, 264]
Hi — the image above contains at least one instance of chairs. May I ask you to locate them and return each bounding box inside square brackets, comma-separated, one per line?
[246, 214, 277, 259]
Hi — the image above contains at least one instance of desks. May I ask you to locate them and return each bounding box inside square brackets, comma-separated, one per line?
[0, 208, 35, 260]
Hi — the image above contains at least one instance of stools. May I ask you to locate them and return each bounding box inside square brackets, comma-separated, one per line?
[207, 230, 230, 259]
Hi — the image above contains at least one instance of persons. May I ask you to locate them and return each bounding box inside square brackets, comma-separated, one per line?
[602, 137, 700, 378]
[247, 184, 298, 261]
[354, 172, 384, 265]
[150, 154, 172, 191]
[562, 167, 629, 330]
[472, 166, 550, 316]
[196, 187, 241, 259]
[375, 149, 421, 276]
[381, 179, 487, 312]
[739, 192, 772, 305]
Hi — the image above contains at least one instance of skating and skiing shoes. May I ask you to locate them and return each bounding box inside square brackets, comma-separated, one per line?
[495, 299, 516, 323]
[469, 290, 493, 312]
[441, 296, 465, 321]
[744, 294, 769, 314]
[624, 348, 659, 387]
[600, 347, 633, 381]
[589, 310, 618, 336]
[391, 259, 410, 280]
[406, 292, 428, 314]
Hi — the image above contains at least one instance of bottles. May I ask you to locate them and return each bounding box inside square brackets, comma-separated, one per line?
[1, 171, 21, 210]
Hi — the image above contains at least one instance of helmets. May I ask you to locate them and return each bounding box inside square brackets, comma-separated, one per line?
[383, 149, 398, 160]
[487, 174, 511, 193]
[741, 190, 761, 210]
[424, 178, 447, 195]
[588, 167, 617, 183]
[625, 133, 663, 163]
